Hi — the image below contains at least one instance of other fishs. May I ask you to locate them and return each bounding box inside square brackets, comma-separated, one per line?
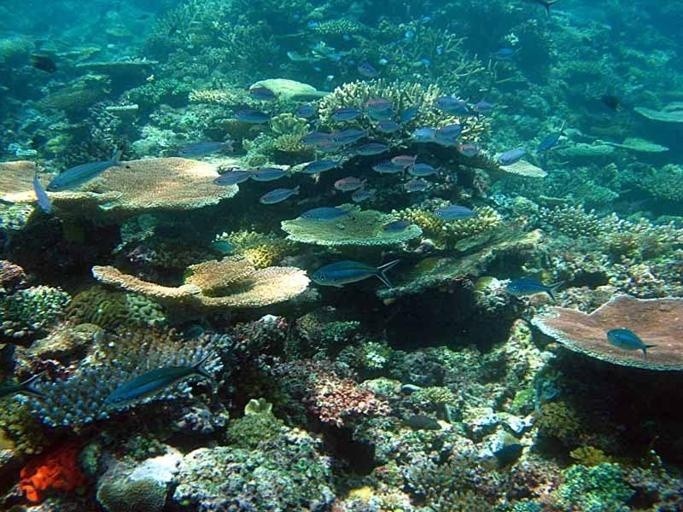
[32, 0, 568, 344]
